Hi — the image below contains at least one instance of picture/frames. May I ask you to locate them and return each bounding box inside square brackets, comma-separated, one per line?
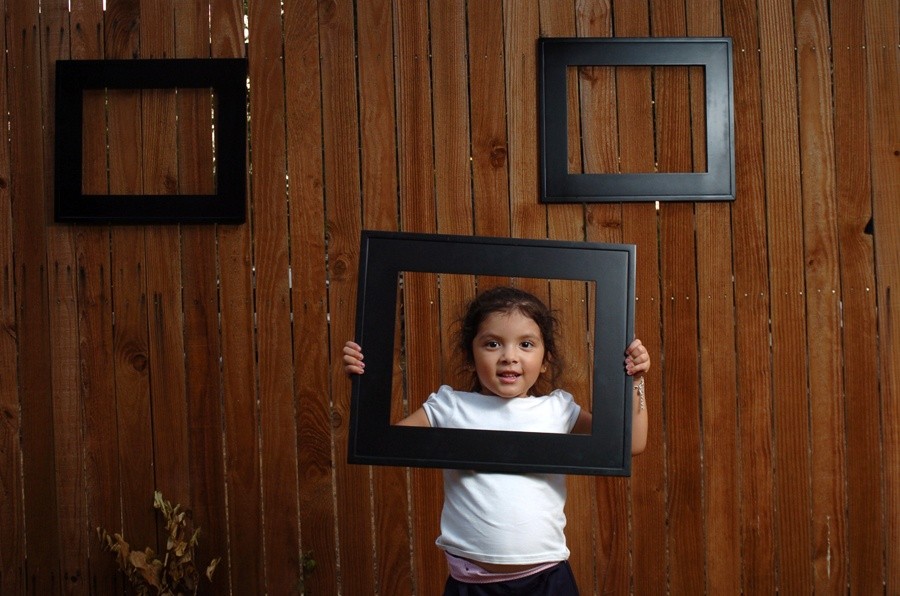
[50, 55, 252, 231]
[344, 227, 641, 482]
[535, 33, 740, 207]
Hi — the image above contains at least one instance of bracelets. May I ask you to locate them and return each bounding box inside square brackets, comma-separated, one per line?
[633, 376, 646, 409]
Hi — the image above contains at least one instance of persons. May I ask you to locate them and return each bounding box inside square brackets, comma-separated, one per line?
[341, 285, 649, 596]
